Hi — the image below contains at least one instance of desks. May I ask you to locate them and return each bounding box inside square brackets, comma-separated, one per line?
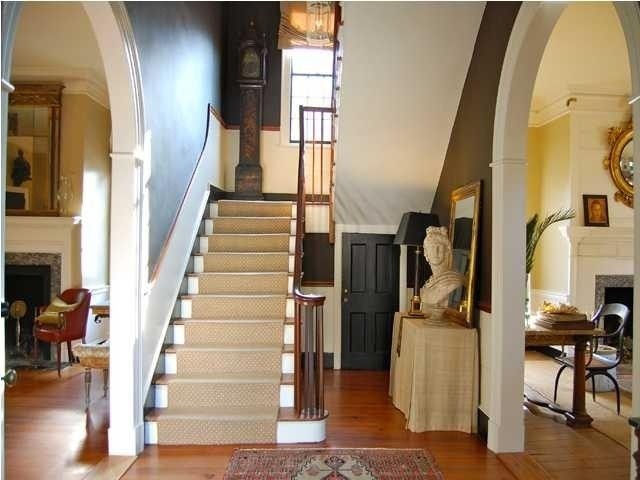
[388, 311, 479, 434]
[523, 322, 602, 429]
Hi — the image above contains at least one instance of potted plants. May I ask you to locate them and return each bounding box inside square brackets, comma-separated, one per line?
[525, 208, 577, 321]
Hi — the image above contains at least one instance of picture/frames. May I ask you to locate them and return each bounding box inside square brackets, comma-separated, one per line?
[582, 194, 610, 227]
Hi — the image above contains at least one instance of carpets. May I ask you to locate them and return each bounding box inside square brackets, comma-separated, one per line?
[222, 447, 445, 480]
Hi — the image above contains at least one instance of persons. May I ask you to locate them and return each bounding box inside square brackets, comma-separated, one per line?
[419, 226, 464, 314]
[590, 200, 605, 222]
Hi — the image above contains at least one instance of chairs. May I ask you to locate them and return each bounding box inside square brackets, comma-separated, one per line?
[553, 303, 630, 416]
[34, 287, 92, 379]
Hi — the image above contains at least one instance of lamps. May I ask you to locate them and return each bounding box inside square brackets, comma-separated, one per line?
[392, 210, 441, 317]
[305, 1, 332, 46]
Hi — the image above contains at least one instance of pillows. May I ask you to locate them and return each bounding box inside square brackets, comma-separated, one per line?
[35, 296, 78, 328]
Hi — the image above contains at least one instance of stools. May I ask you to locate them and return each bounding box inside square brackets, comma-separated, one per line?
[74, 335, 109, 411]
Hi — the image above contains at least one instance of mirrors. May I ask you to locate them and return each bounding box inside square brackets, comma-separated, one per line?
[5, 82, 66, 216]
[444, 179, 482, 328]
